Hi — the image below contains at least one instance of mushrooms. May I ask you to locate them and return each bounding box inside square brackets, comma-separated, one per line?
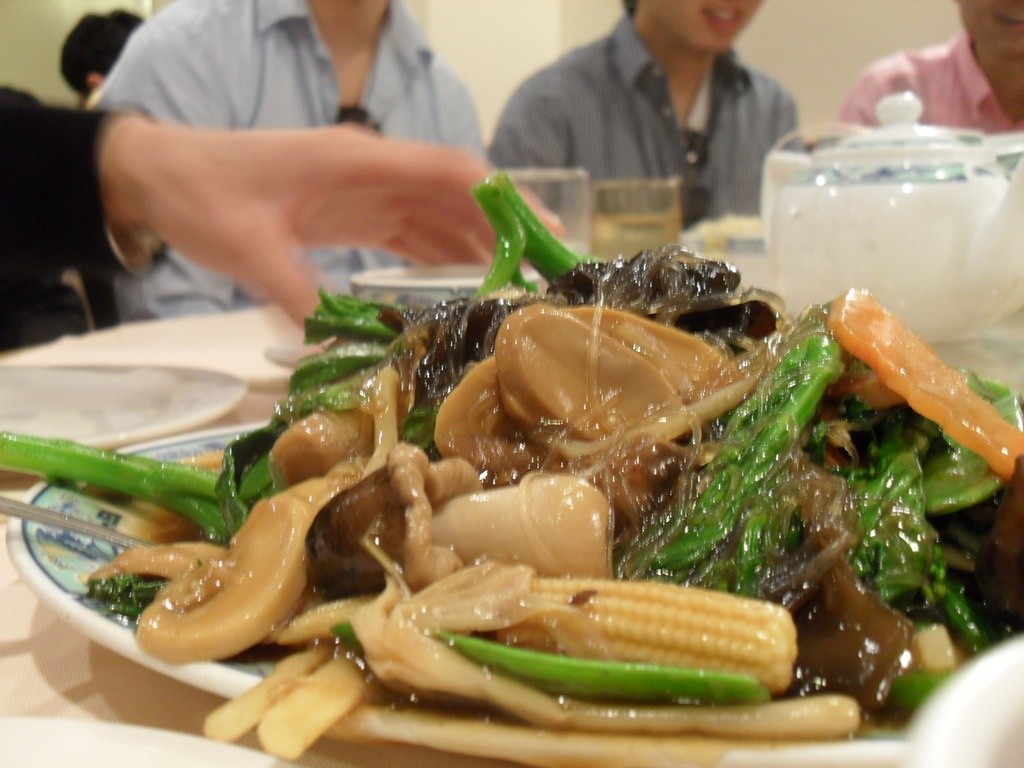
[87, 303, 739, 750]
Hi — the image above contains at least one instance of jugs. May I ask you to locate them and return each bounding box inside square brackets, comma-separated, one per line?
[750, 91, 1024, 337]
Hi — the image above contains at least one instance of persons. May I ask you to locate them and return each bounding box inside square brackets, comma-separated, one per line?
[830, 0, 1023, 139]
[2, 79, 569, 360]
[55, 8, 146, 114]
[75, 1, 490, 337]
[490, 0, 802, 257]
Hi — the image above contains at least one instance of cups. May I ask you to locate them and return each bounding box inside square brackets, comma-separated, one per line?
[509, 165, 592, 262]
[594, 179, 681, 263]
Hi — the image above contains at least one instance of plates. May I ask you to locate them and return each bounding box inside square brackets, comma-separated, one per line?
[16, 419, 905, 763]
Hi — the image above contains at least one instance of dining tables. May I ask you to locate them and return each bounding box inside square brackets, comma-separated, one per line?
[0, 299, 1024, 768]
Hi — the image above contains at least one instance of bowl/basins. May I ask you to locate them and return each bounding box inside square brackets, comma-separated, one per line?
[348, 262, 534, 311]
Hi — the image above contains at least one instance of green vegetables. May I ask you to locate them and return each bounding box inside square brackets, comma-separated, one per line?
[0, 166, 615, 616]
[637, 294, 1021, 729]
